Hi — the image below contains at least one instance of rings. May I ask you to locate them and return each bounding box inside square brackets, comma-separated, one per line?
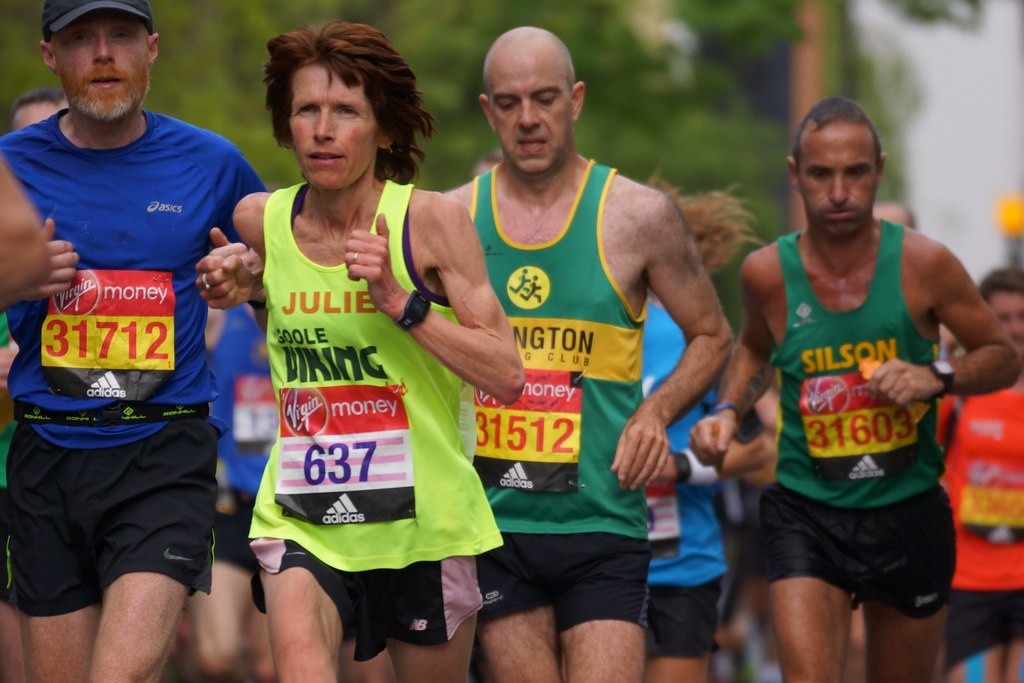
[202, 272, 212, 290]
[352, 252, 359, 262]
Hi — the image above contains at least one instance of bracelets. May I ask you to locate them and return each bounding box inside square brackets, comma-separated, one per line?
[707, 402, 740, 420]
[247, 299, 266, 311]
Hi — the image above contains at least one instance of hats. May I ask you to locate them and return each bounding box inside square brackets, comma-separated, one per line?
[41, 0, 154, 42]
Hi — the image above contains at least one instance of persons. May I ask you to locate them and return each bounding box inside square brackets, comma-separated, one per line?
[435, 23, 780, 683]
[195, 23, 524, 682]
[0, 0, 275, 683]
[933, 268, 1024, 683]
[689, 97, 1023, 683]
[872, 201, 915, 230]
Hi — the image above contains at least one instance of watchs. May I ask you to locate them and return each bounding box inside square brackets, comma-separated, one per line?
[931, 360, 955, 390]
[395, 287, 432, 331]
